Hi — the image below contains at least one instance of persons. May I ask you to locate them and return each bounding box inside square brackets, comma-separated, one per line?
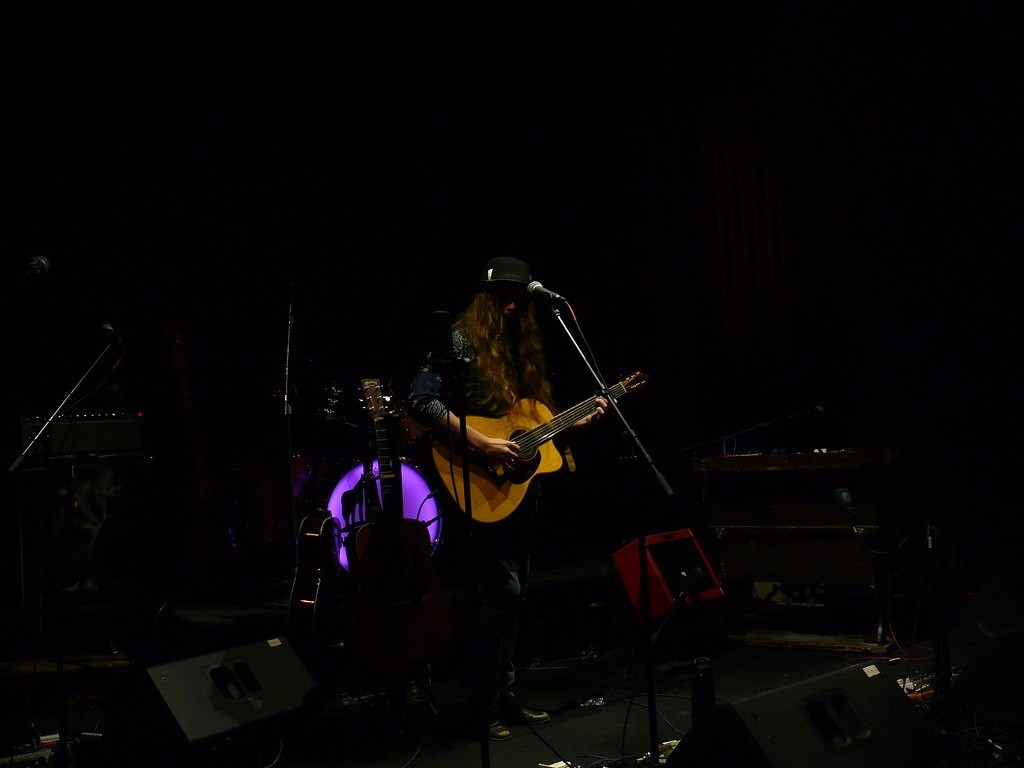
[404, 256, 548, 742]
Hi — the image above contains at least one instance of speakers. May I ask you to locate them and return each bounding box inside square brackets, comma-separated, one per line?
[666, 660, 947, 767]
[140, 635, 325, 753]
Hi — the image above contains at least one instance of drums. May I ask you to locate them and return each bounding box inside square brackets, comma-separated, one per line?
[388, 387, 451, 451]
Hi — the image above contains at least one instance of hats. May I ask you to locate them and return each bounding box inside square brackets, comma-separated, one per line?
[480, 257, 530, 285]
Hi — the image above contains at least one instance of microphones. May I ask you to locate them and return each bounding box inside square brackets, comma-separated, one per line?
[102, 322, 125, 346]
[526, 281, 566, 307]
[431, 306, 454, 356]
[770, 405, 825, 430]
[287, 280, 295, 308]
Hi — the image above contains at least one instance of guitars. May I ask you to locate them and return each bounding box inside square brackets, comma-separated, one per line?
[347, 378, 456, 666]
[284, 384, 348, 660]
[431, 371, 654, 523]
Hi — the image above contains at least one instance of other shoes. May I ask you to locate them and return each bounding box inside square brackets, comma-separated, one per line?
[502, 706, 550, 726]
[488, 719, 512, 741]
